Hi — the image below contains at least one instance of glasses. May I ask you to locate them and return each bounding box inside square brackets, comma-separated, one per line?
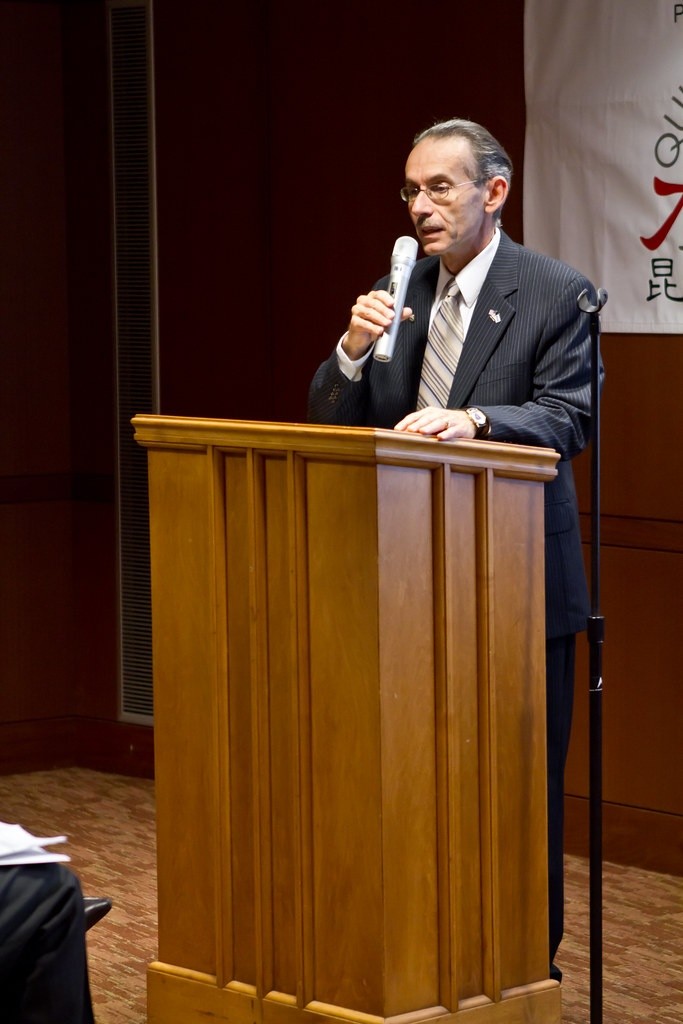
[400, 176, 485, 202]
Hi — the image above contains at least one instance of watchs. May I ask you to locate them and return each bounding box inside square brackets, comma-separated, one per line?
[463, 407, 487, 439]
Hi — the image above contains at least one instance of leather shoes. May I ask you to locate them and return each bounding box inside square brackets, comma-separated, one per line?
[87, 895, 110, 930]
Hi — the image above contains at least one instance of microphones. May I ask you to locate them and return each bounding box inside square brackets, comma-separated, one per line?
[373, 236, 418, 363]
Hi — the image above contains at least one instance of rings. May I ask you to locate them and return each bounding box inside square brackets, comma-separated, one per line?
[441, 417, 451, 428]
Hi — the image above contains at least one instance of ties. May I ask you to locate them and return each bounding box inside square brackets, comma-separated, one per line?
[417, 278, 464, 414]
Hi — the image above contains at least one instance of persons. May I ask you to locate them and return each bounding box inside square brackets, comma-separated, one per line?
[0, 859, 113, 1024]
[308, 117, 606, 988]
